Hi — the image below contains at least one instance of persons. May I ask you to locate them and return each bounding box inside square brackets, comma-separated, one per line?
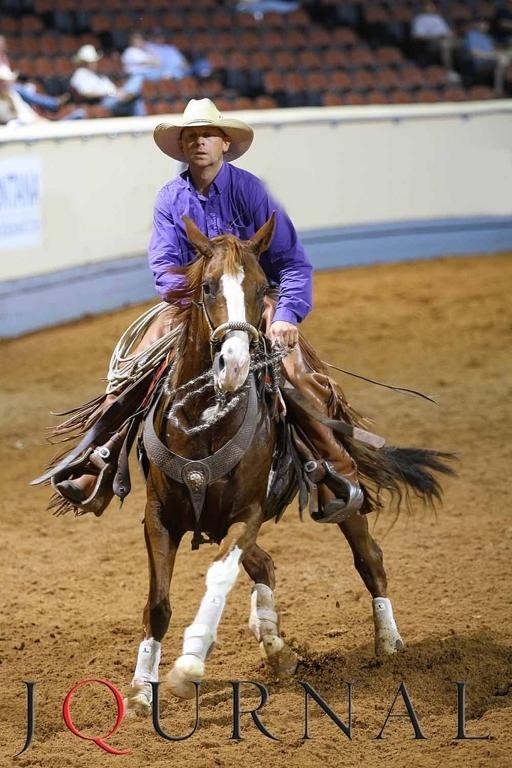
[0, 25, 188, 126]
[409, 0, 512, 97]
[56, 97, 361, 517]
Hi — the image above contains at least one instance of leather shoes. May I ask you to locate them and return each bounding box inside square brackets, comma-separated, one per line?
[319, 464, 373, 516]
[54, 465, 112, 515]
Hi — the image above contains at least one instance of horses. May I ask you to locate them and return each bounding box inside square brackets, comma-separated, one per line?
[122, 211, 456, 717]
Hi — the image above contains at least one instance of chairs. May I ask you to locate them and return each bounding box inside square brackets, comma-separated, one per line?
[0, 0, 512, 123]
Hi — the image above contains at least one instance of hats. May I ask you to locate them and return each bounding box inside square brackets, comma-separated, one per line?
[0, 65, 20, 83]
[73, 44, 102, 63]
[153, 96, 253, 162]
